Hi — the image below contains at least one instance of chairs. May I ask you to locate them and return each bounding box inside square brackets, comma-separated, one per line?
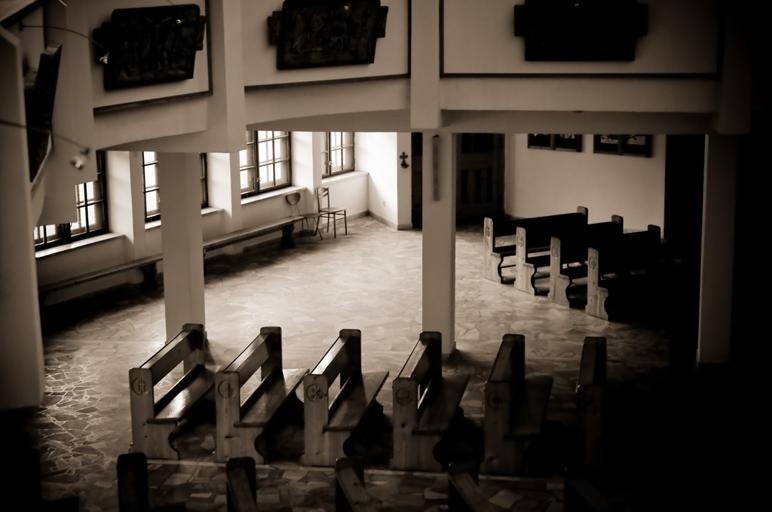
[313, 187, 347, 238]
[286, 193, 323, 241]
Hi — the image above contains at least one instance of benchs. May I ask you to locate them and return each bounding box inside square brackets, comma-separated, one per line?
[484, 206, 664, 321]
[39, 215, 310, 311]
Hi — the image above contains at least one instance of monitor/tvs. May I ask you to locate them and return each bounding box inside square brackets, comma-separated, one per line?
[272, 1, 379, 72]
[520, 0, 639, 64]
[26, 42, 64, 182]
[103, 3, 199, 89]
